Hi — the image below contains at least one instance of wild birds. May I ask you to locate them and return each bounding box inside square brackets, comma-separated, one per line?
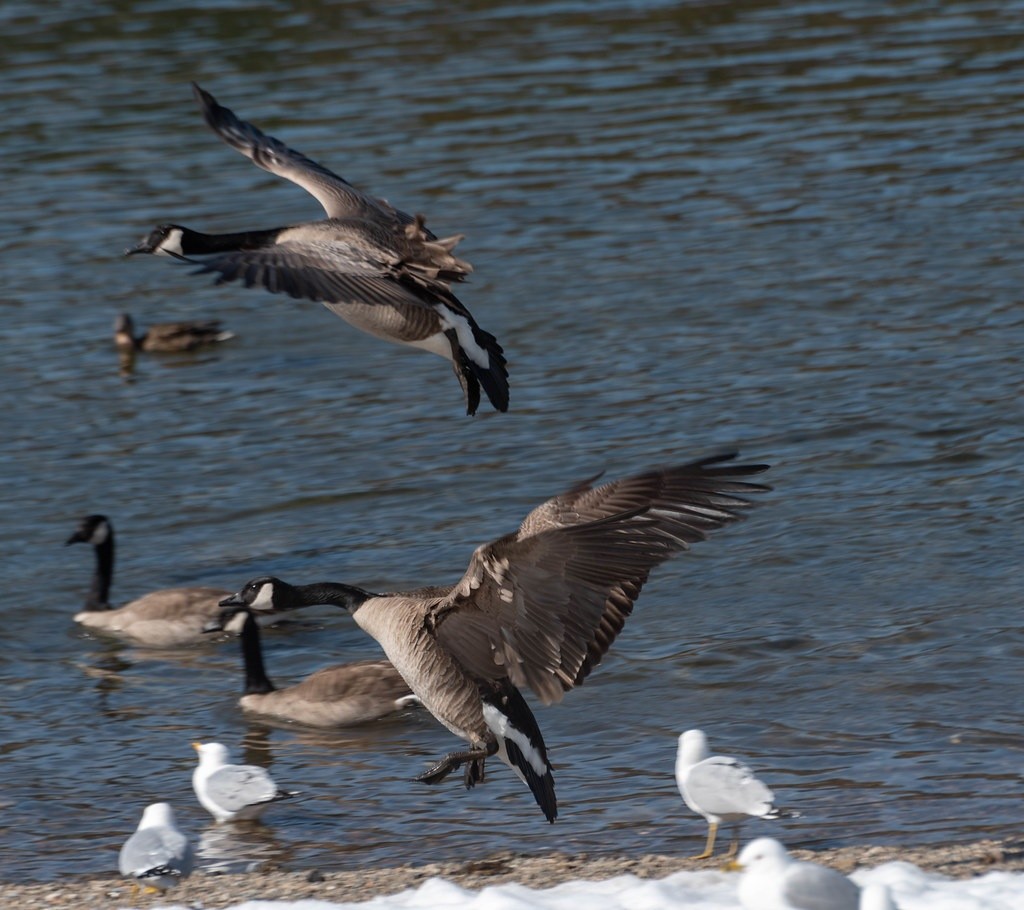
[220, 446, 774, 823]
[675, 729, 776, 861]
[729, 837, 860, 910]
[64, 514, 237, 647]
[200, 604, 420, 726]
[119, 802, 186, 877]
[114, 311, 237, 376]
[192, 742, 275, 824]
[122, 84, 508, 420]
